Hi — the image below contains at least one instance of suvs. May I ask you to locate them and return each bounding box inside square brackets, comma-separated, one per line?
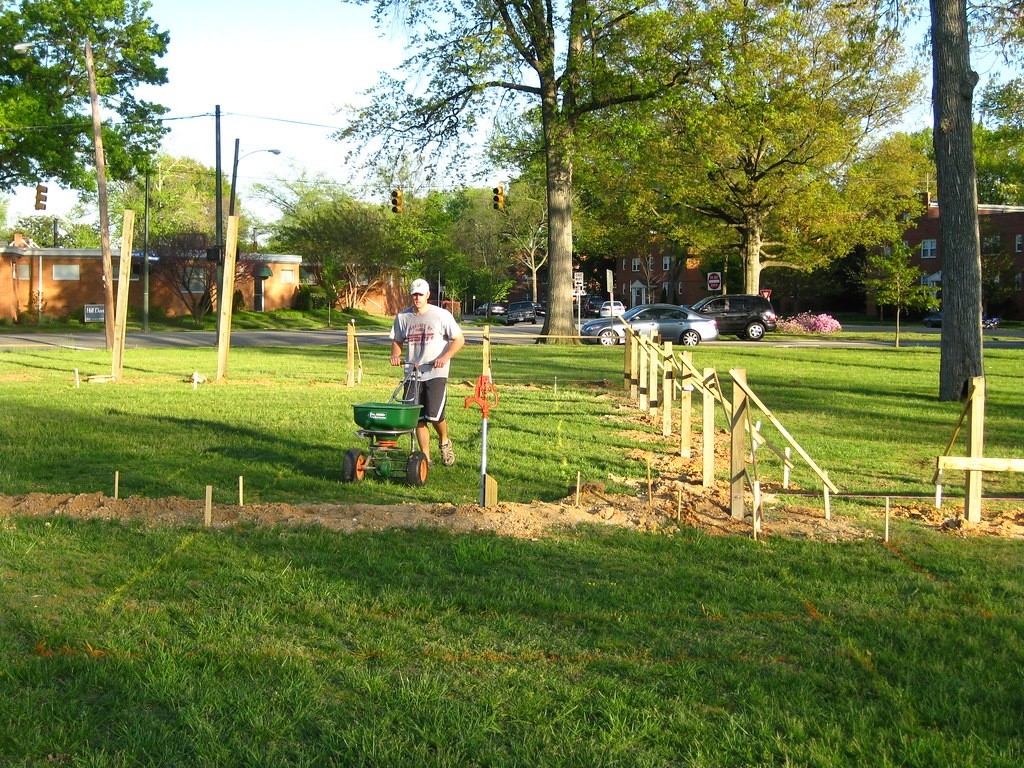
[657, 293, 778, 342]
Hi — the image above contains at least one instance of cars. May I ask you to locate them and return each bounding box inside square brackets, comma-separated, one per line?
[475, 303, 506, 316]
[573, 296, 604, 318]
[923, 311, 999, 330]
[600, 301, 627, 317]
[507, 301, 538, 325]
[579, 303, 720, 346]
[535, 307, 547, 316]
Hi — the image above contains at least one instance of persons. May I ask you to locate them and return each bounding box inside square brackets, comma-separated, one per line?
[389, 278, 465, 468]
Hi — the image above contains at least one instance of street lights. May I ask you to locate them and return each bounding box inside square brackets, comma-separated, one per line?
[228, 137, 281, 320]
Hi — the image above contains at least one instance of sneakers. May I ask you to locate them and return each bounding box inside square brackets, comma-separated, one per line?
[428, 460, 432, 469]
[439, 438, 455, 466]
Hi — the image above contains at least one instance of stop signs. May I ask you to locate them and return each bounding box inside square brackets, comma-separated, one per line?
[707, 272, 722, 292]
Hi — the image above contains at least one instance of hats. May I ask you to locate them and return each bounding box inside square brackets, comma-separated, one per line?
[409, 279, 429, 296]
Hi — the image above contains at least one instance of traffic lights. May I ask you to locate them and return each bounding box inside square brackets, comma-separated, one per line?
[148, 263, 155, 275]
[391, 189, 403, 215]
[134, 261, 144, 275]
[206, 247, 222, 264]
[35, 184, 49, 211]
[494, 185, 505, 212]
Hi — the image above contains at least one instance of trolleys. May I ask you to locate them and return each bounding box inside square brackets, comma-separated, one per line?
[340, 361, 439, 488]
